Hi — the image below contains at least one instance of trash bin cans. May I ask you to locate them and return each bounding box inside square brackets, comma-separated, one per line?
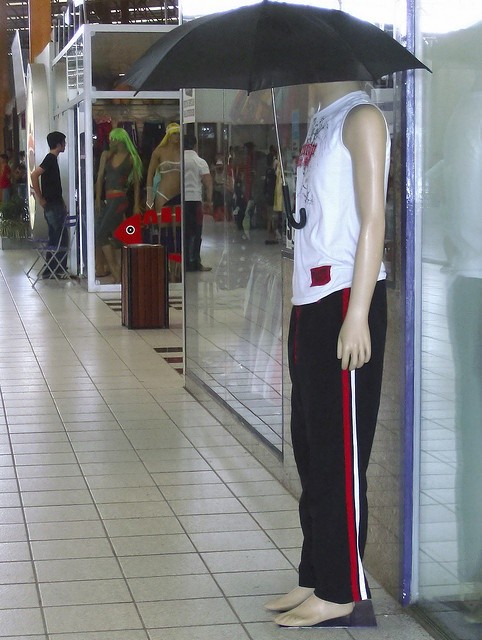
[122, 244, 169, 328]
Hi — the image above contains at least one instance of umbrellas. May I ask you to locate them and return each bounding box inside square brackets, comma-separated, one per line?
[110, 0, 432, 226]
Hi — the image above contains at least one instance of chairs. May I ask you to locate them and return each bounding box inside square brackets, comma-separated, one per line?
[27, 213, 77, 285]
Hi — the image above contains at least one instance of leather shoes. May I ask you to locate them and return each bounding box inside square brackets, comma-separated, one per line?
[199, 266, 212, 272]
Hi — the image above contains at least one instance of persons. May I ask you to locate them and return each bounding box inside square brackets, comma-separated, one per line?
[94, 128, 139, 288]
[31, 131, 70, 279]
[0, 153, 13, 203]
[14, 151, 26, 181]
[266, 82, 394, 628]
[5, 148, 15, 182]
[184, 135, 213, 272]
[150, 121, 181, 232]
